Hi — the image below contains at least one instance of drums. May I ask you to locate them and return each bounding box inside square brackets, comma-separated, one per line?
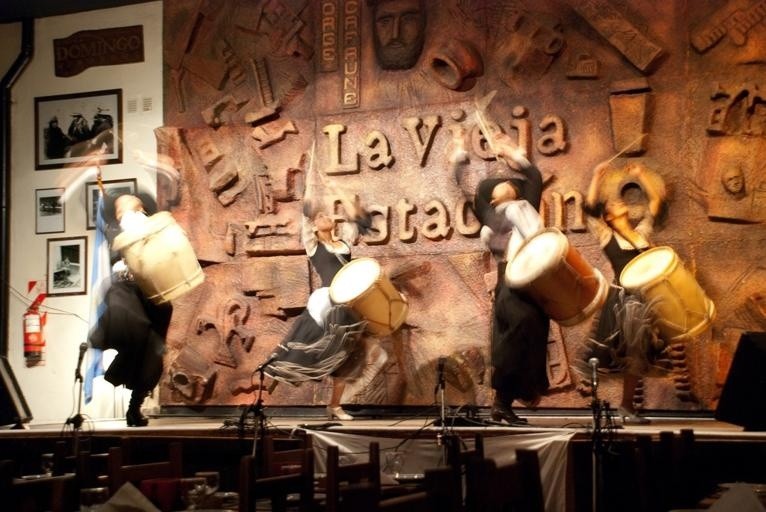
[329, 257, 409, 337]
[112, 210, 205, 305]
[620, 246, 717, 341]
[505, 228, 608, 326]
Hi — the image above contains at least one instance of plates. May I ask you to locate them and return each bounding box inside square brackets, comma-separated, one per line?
[394, 474, 423, 483]
[21, 475, 40, 479]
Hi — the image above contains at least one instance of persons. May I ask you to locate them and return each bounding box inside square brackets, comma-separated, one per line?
[447, 122, 548, 425]
[260, 174, 374, 422]
[44, 107, 113, 159]
[89, 157, 175, 430]
[366, 0, 424, 72]
[575, 161, 667, 424]
[722, 165, 746, 200]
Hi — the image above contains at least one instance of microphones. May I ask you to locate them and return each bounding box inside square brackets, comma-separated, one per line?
[76, 343, 88, 375]
[255, 353, 279, 372]
[438, 354, 447, 384]
[588, 357, 600, 391]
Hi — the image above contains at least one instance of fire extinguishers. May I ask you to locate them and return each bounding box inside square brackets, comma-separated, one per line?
[23, 294, 47, 357]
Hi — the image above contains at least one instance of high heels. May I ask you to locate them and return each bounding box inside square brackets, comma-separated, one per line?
[126, 409, 149, 427]
[326, 405, 354, 421]
[491, 406, 528, 423]
[618, 404, 651, 424]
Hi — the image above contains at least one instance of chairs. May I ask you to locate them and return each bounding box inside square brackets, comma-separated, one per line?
[0, 426, 705, 512]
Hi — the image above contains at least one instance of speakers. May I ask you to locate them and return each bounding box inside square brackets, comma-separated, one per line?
[715, 331, 766, 432]
[0, 355, 34, 426]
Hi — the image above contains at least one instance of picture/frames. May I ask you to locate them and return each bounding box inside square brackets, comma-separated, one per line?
[34, 89, 122, 171]
[84, 177, 137, 230]
[45, 236, 86, 296]
[34, 184, 67, 235]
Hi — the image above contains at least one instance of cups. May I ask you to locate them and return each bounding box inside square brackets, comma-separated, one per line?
[43, 452, 56, 476]
[386, 452, 401, 476]
[195, 471, 220, 496]
[181, 478, 206, 509]
